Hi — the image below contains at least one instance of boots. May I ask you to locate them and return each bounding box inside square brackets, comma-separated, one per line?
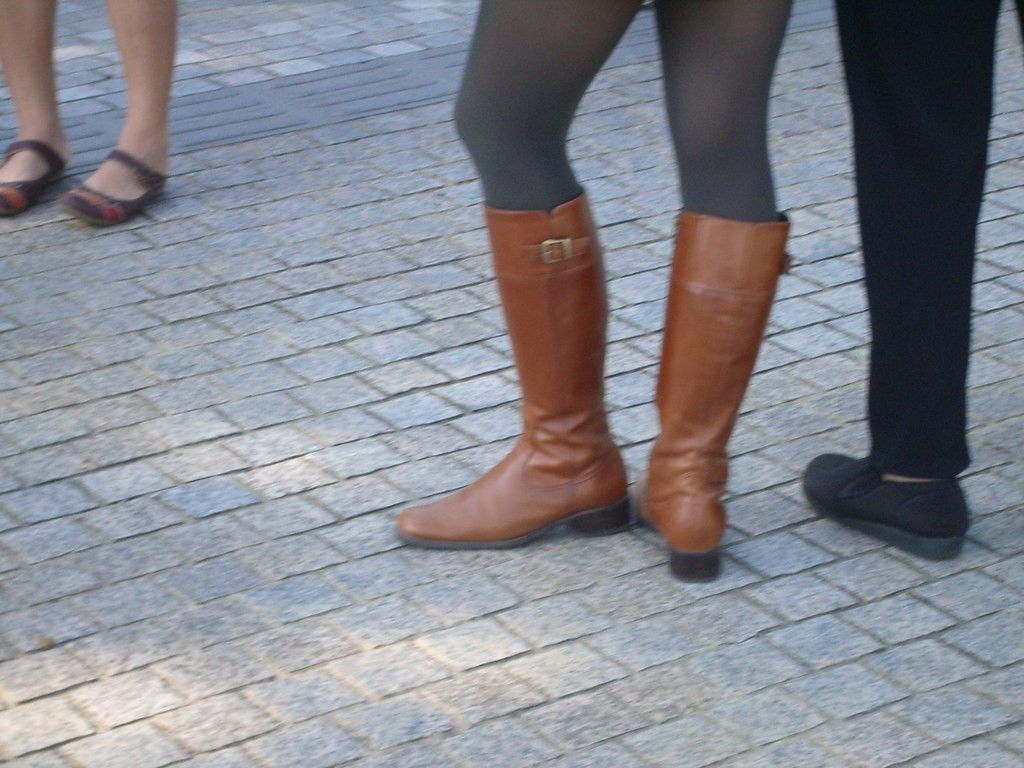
[398, 188, 630, 551]
[635, 205, 791, 580]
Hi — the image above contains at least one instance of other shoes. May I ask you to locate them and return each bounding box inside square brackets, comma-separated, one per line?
[0, 133, 72, 218]
[66, 146, 169, 228]
[802, 451, 966, 559]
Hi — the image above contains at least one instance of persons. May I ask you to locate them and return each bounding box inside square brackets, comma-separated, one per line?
[397, 0, 1024, 581]
[1, 0, 178, 227]
